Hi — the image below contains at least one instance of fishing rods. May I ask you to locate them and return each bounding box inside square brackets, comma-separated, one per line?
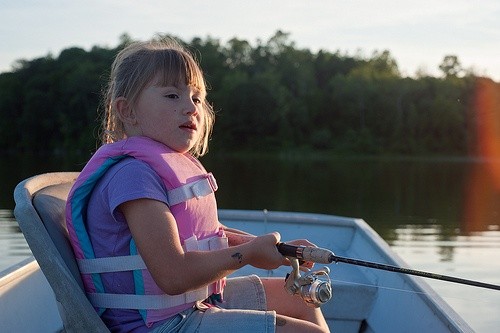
[222, 231, 500, 307]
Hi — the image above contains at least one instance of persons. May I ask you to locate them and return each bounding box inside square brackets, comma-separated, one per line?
[65, 42, 335, 333]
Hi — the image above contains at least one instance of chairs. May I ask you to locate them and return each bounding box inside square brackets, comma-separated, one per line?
[12, 172, 113, 333]
